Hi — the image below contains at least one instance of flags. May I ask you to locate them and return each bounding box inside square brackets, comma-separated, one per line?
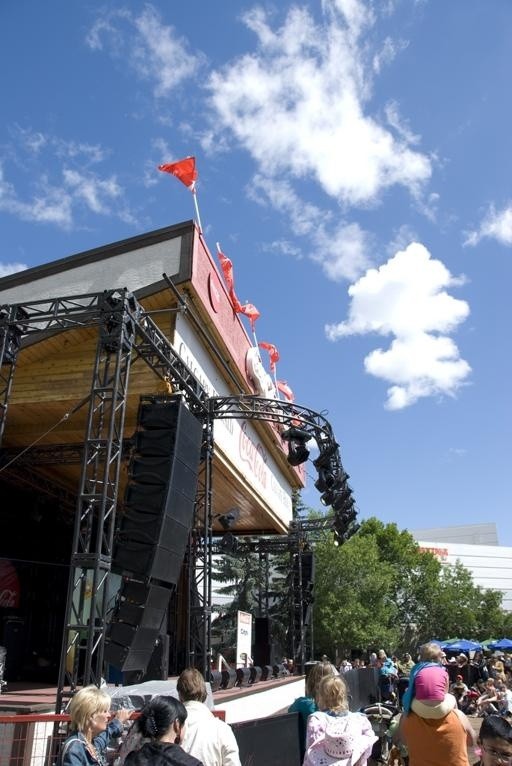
[157, 155, 199, 191]
[277, 380, 294, 400]
[218, 252, 260, 330]
[258, 343, 279, 369]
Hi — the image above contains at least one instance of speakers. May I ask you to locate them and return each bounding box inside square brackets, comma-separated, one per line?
[236, 668, 251, 687]
[221, 670, 237, 690]
[286, 552, 316, 646]
[3, 614, 27, 682]
[104, 393, 205, 672]
[262, 665, 273, 681]
[250, 666, 261, 683]
[274, 664, 284, 677]
[210, 671, 222, 692]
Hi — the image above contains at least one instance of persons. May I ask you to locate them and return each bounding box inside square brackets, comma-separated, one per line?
[172, 668, 243, 766]
[0, 556, 20, 692]
[293, 633, 510, 766]
[55, 685, 137, 766]
[121, 696, 208, 765]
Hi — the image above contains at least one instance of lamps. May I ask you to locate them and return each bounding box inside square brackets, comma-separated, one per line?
[283, 427, 359, 544]
[207, 663, 286, 693]
